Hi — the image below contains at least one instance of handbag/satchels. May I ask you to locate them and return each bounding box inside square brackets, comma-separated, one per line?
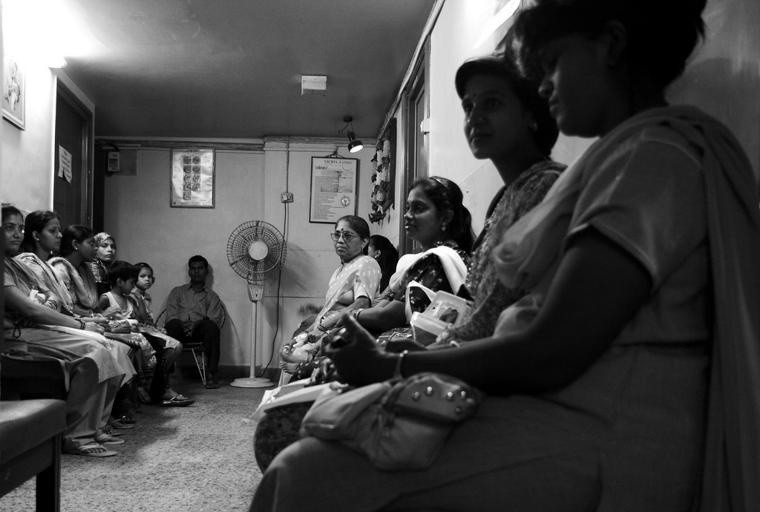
[302, 332, 481, 470]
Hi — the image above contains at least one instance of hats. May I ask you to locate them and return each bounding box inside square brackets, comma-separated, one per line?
[455, 53, 559, 150]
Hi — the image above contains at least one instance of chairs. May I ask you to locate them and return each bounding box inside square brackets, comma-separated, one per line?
[154, 304, 225, 386]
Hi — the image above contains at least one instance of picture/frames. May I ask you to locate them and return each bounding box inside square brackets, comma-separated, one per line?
[307, 155, 361, 224]
[1, 48, 26, 131]
[168, 146, 216, 209]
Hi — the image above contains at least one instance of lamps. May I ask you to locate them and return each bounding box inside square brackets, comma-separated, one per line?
[342, 116, 363, 153]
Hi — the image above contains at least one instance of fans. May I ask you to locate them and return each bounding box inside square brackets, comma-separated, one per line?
[228, 221, 288, 389]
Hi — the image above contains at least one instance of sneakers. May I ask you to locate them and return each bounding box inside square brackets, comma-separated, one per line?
[205, 376, 219, 388]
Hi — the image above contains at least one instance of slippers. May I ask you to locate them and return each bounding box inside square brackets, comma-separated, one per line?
[96, 433, 124, 444]
[105, 425, 122, 435]
[117, 411, 137, 423]
[163, 389, 196, 406]
[112, 419, 134, 428]
[73, 442, 118, 456]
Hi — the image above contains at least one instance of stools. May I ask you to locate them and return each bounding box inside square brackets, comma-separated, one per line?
[0, 399, 66, 512]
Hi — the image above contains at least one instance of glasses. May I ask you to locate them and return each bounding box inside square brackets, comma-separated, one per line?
[331, 233, 354, 241]
[403, 204, 426, 214]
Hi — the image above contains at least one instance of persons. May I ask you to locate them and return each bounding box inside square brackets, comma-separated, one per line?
[246, 0, 760, 512]
[164, 253, 226, 391]
[1, 203, 195, 459]
